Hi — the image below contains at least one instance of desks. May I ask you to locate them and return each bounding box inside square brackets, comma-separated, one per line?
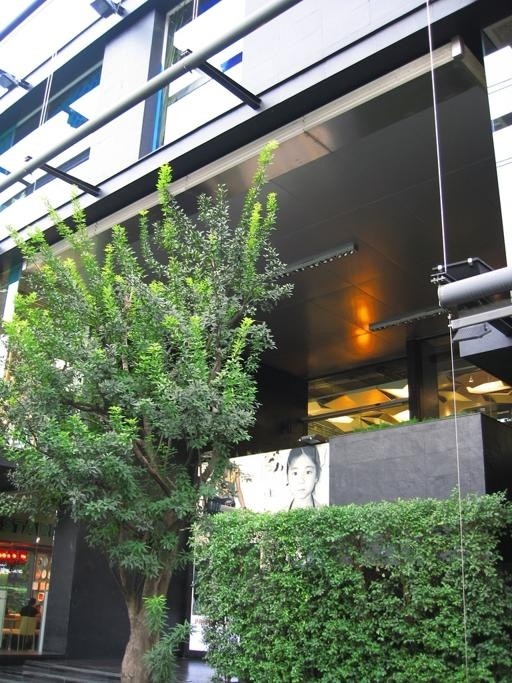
[4, 614, 21, 650]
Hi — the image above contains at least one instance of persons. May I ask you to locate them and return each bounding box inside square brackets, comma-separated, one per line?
[235, 446, 323, 556]
[20, 598, 40, 616]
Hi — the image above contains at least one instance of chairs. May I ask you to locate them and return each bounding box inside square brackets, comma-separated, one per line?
[9, 616, 37, 652]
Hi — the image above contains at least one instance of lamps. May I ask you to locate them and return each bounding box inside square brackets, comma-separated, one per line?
[0, 68, 30, 91]
[90, 1, 125, 20]
